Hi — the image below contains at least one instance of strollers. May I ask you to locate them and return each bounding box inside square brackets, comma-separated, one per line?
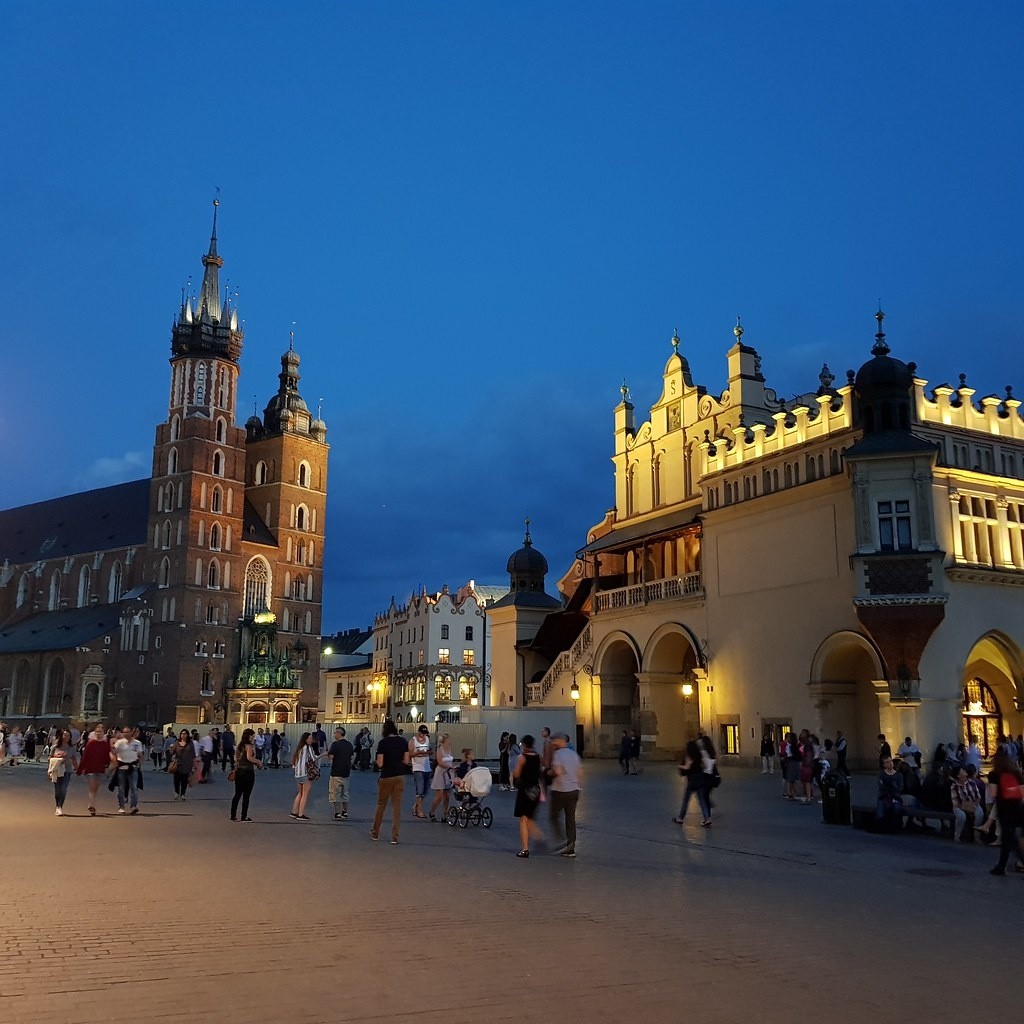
[447, 766, 493, 829]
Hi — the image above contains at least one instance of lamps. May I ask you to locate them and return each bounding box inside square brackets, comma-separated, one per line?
[571, 675, 580, 701]
[682, 672, 693, 703]
[897, 651, 913, 703]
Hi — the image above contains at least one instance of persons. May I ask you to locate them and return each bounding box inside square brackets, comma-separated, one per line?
[429, 733, 456, 824]
[108, 727, 144, 814]
[427, 734, 436, 779]
[409, 725, 433, 818]
[671, 728, 848, 827]
[288, 733, 328, 821]
[47, 728, 79, 816]
[324, 727, 354, 821]
[77, 724, 115, 816]
[454, 748, 477, 792]
[499, 732, 511, 792]
[539, 727, 583, 858]
[230, 728, 263, 823]
[169, 728, 196, 800]
[874, 732, 1024, 878]
[398, 729, 404, 738]
[368, 719, 409, 845]
[508, 734, 521, 792]
[619, 729, 640, 775]
[760, 732, 775, 774]
[513, 735, 543, 858]
[0, 721, 375, 783]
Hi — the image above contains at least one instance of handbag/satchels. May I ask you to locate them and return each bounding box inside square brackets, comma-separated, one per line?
[961, 800, 976, 813]
[306, 744, 320, 781]
[227, 771, 235, 782]
[539, 784, 547, 803]
[712, 764, 721, 788]
[167, 762, 179, 774]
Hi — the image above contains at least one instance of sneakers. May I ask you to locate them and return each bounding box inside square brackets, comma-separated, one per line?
[289, 814, 311, 821]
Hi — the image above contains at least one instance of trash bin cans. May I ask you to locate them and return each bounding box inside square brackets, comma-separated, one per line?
[820, 771, 850, 825]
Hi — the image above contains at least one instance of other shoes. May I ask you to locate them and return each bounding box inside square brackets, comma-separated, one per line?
[673, 817, 712, 828]
[352, 763, 372, 772]
[429, 811, 438, 822]
[230, 818, 253, 823]
[517, 850, 529, 859]
[54, 807, 139, 817]
[369, 830, 397, 845]
[906, 819, 1002, 847]
[760, 770, 774, 774]
[623, 771, 637, 776]
[441, 817, 452, 822]
[783, 793, 823, 805]
[416, 808, 427, 818]
[533, 838, 548, 849]
[174, 792, 186, 800]
[989, 868, 1005, 875]
[412, 806, 418, 816]
[333, 812, 348, 821]
[549, 840, 577, 858]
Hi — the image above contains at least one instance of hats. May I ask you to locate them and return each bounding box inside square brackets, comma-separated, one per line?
[419, 725, 429, 734]
[550, 732, 567, 739]
